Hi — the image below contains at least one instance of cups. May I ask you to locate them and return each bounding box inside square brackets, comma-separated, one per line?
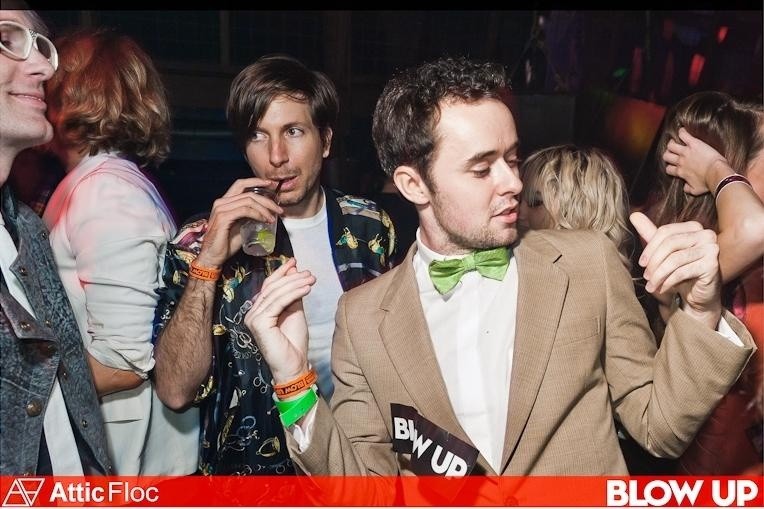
[241, 188, 279, 257]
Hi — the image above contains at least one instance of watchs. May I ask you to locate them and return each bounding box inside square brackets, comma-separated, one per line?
[271, 383, 322, 428]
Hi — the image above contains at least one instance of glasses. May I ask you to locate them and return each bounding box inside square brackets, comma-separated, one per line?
[0, 21, 60, 72]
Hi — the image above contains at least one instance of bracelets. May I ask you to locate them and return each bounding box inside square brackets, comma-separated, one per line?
[713, 173, 752, 205]
[270, 362, 318, 400]
[188, 257, 222, 282]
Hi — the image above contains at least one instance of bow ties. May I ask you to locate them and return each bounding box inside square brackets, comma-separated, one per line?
[429, 248, 511, 296]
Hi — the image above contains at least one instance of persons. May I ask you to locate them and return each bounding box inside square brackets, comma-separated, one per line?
[34, 25, 200, 507]
[0, 11, 114, 507]
[624, 89, 764, 476]
[515, 145, 633, 270]
[245, 56, 757, 508]
[151, 57, 402, 507]
